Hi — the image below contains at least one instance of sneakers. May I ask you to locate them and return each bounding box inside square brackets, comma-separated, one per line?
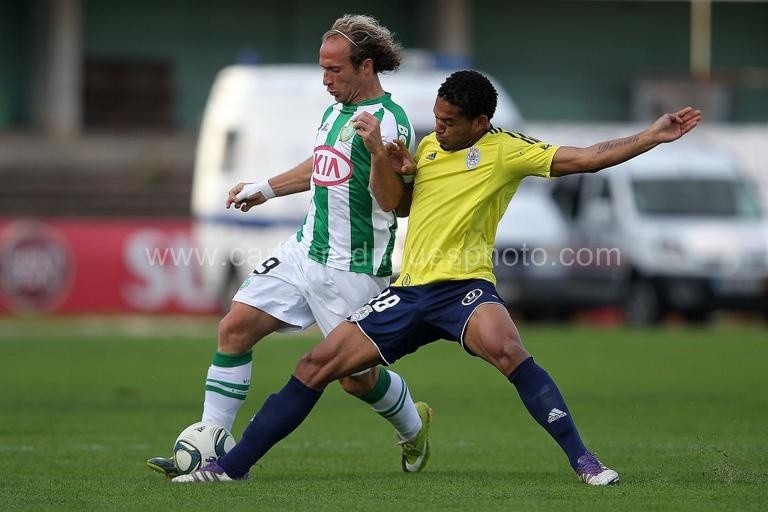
[172, 456, 250, 482]
[400, 401, 431, 474]
[146, 456, 179, 476]
[578, 451, 620, 485]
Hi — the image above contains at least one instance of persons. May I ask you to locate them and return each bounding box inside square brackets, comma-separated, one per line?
[146, 13, 432, 476]
[172, 70, 702, 488]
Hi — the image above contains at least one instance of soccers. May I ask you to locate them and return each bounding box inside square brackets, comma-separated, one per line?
[174, 422, 237, 475]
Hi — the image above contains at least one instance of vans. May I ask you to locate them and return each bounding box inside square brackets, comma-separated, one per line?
[559, 137, 768, 323]
[188, 65, 575, 328]
